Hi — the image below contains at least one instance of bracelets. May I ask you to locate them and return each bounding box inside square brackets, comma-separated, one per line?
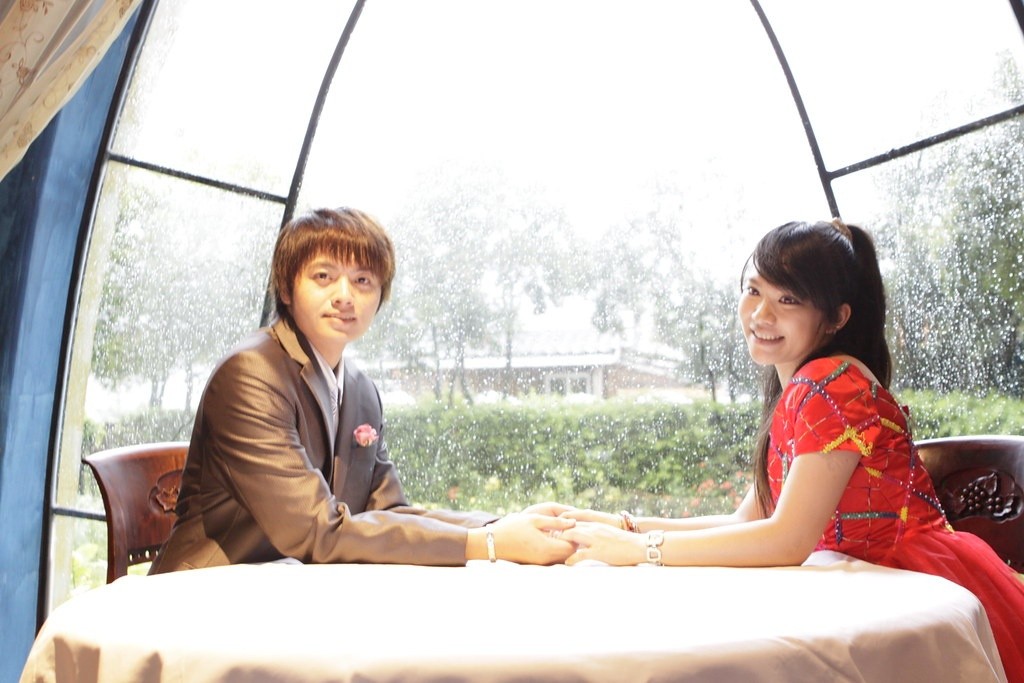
[484, 522, 497, 563]
[645, 528, 669, 567]
[618, 508, 643, 533]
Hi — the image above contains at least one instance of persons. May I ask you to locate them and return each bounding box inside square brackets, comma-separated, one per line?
[551, 216, 970, 596]
[146, 208, 576, 577]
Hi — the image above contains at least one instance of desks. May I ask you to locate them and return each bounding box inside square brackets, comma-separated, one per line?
[18, 551, 1008, 683]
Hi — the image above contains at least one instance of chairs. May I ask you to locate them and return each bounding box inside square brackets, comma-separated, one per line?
[914, 435, 1024, 572]
[81, 441, 190, 584]
